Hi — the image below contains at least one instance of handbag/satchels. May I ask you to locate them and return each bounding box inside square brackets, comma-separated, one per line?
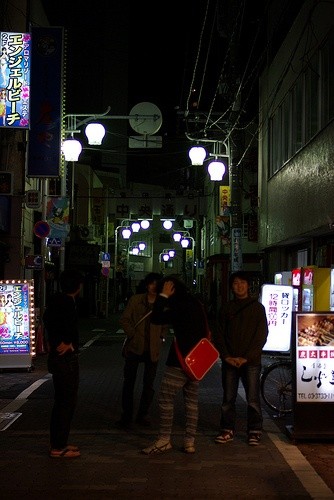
[182, 338, 222, 382]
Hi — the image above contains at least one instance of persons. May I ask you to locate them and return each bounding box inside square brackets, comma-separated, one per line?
[141, 276, 210, 455]
[0, 293, 16, 309]
[116, 273, 167, 430]
[42, 269, 85, 458]
[214, 270, 268, 447]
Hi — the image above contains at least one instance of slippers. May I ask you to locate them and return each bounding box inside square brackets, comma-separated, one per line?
[50, 449, 81, 459]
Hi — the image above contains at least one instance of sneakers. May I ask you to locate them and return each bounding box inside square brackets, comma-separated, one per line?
[246, 432, 261, 445]
[215, 429, 236, 443]
[142, 442, 173, 455]
[183, 441, 195, 453]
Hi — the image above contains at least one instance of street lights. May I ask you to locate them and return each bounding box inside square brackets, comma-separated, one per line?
[57, 106, 112, 294]
[183, 131, 234, 305]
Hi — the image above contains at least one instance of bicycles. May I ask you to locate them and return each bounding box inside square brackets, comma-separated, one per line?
[260, 356, 295, 418]
